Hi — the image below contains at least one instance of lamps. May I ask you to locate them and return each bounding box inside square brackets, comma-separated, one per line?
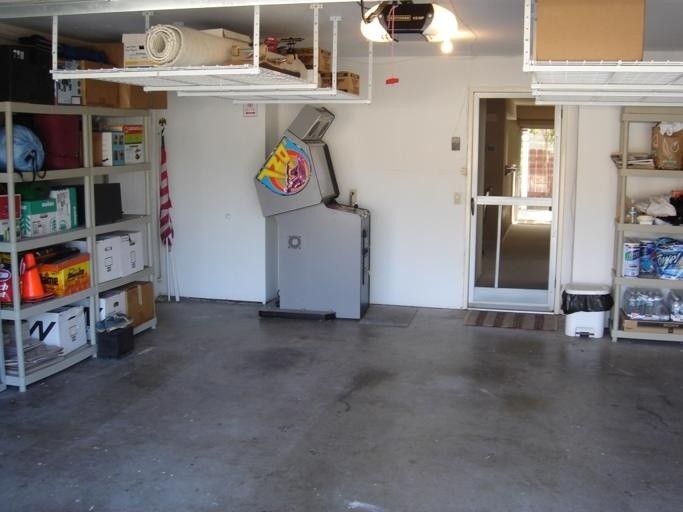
[357, 0, 457, 43]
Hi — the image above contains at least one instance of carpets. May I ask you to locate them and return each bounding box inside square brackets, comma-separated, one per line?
[462, 309, 560, 332]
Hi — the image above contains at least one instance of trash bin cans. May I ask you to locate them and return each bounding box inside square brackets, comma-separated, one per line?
[561, 283, 610, 339]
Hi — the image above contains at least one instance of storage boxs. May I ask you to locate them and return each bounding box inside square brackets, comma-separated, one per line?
[321, 72, 360, 97]
[534, 0, 646, 63]
[199, 28, 252, 46]
[652, 121, 683, 170]
[279, 48, 332, 72]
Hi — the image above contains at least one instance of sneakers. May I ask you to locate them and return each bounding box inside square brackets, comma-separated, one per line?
[96, 313, 134, 333]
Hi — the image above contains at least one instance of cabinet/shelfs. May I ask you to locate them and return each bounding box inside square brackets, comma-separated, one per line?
[0, 101, 157, 392]
[608, 106, 683, 345]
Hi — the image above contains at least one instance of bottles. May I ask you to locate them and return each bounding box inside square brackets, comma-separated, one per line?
[628, 200, 637, 225]
[623, 286, 682, 317]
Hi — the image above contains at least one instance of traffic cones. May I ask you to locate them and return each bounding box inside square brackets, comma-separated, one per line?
[15, 251, 55, 303]
